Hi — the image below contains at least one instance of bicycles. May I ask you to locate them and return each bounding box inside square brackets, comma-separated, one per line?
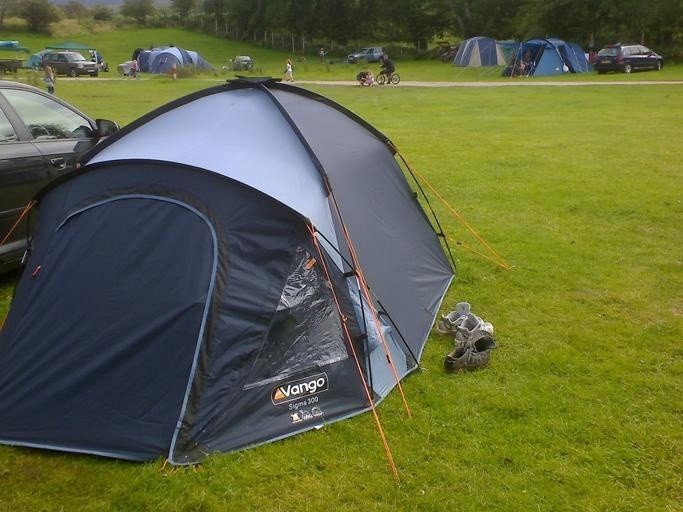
[376, 68, 400, 85]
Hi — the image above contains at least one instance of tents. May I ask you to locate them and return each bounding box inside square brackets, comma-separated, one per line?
[453, 37, 506, 67]
[25, 49, 55, 68]
[1, 72, 457, 467]
[501, 36, 592, 77]
[138, 46, 216, 75]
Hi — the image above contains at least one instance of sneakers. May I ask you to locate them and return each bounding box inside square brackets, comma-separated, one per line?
[436, 301, 496, 375]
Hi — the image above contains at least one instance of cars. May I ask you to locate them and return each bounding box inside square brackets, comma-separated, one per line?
[593, 42, 664, 73]
[347, 47, 384, 64]
[0, 81, 120, 276]
[233, 55, 254, 71]
[42, 51, 98, 77]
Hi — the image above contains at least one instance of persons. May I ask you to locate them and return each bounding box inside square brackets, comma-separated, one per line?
[128, 59, 137, 78]
[286, 59, 294, 82]
[89, 50, 98, 62]
[43, 65, 55, 94]
[505, 51, 533, 76]
[366, 70, 374, 86]
[320, 47, 324, 63]
[380, 55, 395, 84]
[356, 70, 368, 88]
